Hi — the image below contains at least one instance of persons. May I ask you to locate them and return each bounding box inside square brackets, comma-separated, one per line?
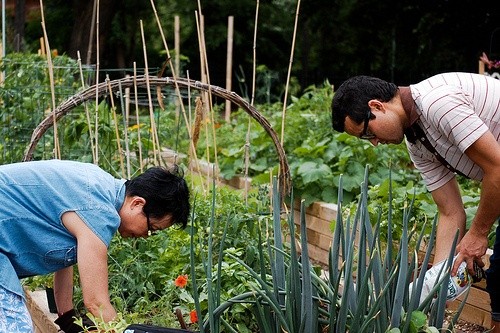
[2, 159, 194, 332]
[330, 71, 500, 333]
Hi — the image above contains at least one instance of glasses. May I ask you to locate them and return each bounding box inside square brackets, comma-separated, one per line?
[357, 110, 376, 140]
[143, 209, 156, 235]
